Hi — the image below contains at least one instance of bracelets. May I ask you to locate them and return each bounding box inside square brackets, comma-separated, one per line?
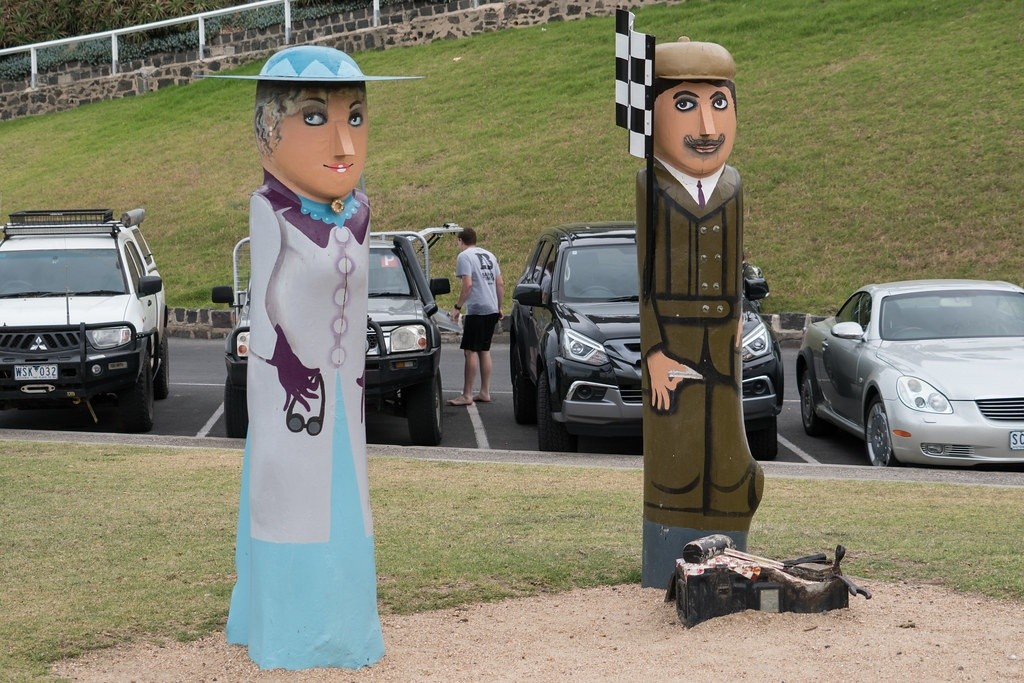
[454, 303, 461, 310]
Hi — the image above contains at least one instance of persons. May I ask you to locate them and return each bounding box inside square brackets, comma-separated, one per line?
[636, 33, 765, 531]
[446, 228, 505, 406]
[198, 47, 425, 671]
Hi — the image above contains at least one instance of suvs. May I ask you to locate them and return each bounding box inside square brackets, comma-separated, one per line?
[212, 223, 464, 447]
[0, 209, 169, 433]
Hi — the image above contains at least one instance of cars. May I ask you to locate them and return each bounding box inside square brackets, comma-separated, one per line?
[510, 221, 784, 461]
[796, 279, 1024, 472]
